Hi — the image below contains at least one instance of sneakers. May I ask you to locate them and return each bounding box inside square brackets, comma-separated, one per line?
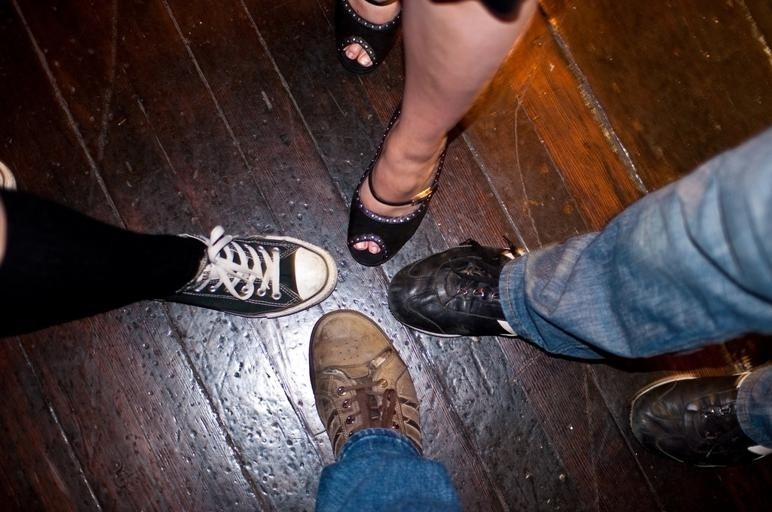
[388, 235, 521, 338]
[629, 371, 772, 469]
[156, 224, 338, 319]
[309, 309, 424, 459]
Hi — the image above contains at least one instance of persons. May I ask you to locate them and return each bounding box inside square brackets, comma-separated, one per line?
[385, 121, 772, 468]
[306, 308, 460, 511]
[341, 1, 537, 265]
[1, 165, 340, 342]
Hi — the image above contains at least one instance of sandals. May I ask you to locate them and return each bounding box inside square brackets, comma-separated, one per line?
[335, 0, 402, 74]
[347, 104, 445, 266]
